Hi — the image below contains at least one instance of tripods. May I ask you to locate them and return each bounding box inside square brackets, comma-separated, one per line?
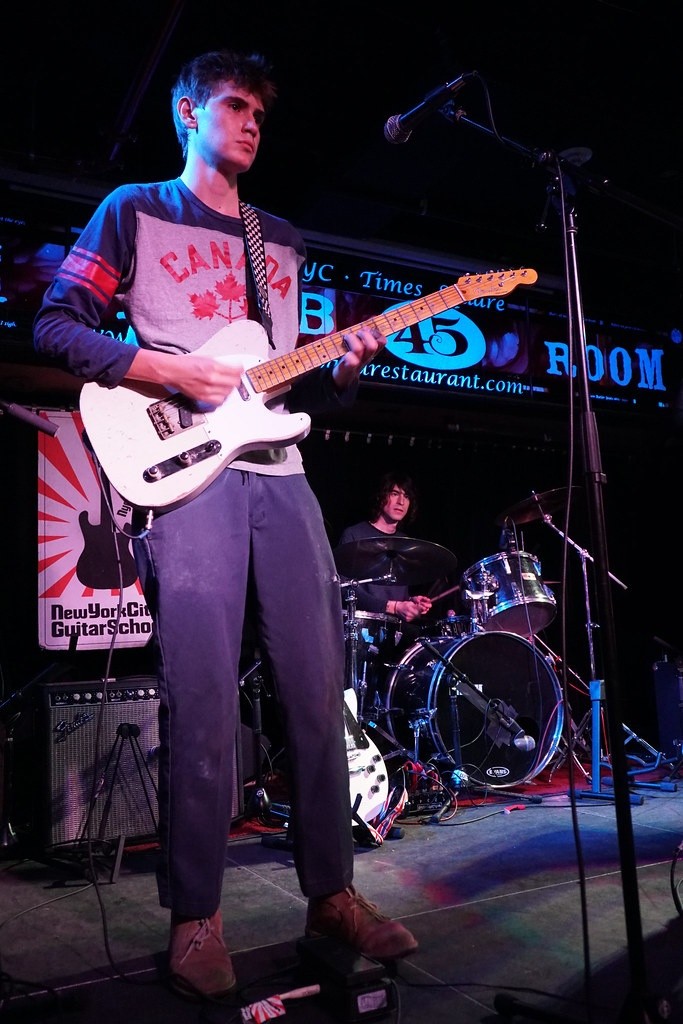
[410, 639, 543, 823]
[230, 656, 290, 828]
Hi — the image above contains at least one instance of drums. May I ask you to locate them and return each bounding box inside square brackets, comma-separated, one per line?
[385, 629, 566, 790]
[421, 613, 474, 638]
[457, 550, 559, 639]
[342, 608, 403, 666]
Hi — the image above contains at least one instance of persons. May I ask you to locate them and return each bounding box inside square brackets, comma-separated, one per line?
[36, 50, 418, 994]
[336, 472, 433, 642]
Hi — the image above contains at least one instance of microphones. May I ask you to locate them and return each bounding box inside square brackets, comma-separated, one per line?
[514, 735, 536, 753]
[383, 72, 476, 144]
[149, 746, 160, 759]
[499, 516, 509, 551]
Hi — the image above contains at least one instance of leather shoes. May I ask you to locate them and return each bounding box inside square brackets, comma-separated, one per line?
[304, 883, 419, 959]
[166, 904, 237, 998]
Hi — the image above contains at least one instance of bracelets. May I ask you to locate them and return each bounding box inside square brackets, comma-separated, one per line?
[394, 601, 397, 614]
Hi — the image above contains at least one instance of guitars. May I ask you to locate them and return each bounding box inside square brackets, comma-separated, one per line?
[78, 263, 540, 516]
[342, 686, 390, 827]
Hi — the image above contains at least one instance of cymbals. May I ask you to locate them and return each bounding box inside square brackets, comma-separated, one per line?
[494, 485, 586, 527]
[332, 536, 459, 586]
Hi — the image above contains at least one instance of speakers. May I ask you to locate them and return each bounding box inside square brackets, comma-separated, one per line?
[33, 675, 161, 853]
